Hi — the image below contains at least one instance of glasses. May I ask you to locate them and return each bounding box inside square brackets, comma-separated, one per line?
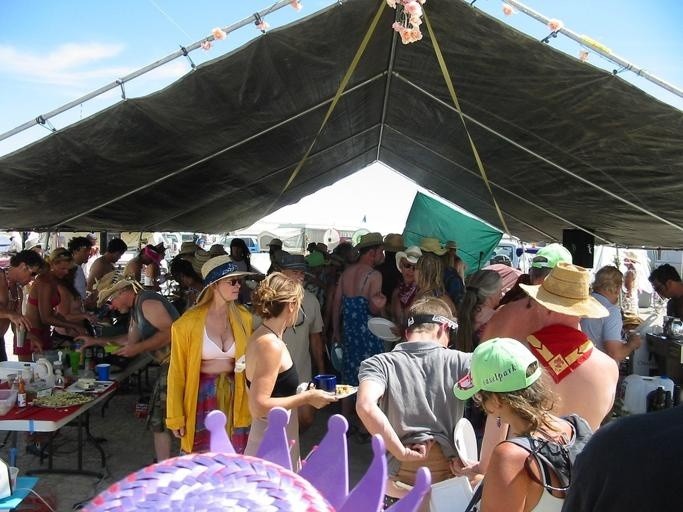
[26, 265, 37, 277]
[104, 293, 117, 305]
[401, 263, 415, 271]
[219, 280, 240, 286]
[52, 252, 70, 262]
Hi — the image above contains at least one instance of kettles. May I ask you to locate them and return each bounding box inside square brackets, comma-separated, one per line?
[32, 357, 54, 388]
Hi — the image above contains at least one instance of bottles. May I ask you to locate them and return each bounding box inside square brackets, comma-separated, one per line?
[16, 363, 32, 407]
[74, 341, 83, 369]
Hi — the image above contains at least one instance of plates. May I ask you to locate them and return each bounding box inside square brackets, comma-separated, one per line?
[366, 316, 401, 343]
[322, 342, 343, 374]
[453, 415, 478, 467]
[30, 350, 66, 365]
[333, 385, 359, 401]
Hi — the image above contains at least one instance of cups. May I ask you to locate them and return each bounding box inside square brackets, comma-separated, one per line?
[95, 363, 114, 380]
[313, 374, 335, 394]
[69, 351, 79, 376]
[6, 373, 16, 386]
[16, 326, 25, 348]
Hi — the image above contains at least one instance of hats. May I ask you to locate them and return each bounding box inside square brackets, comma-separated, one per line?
[78, 452, 335, 512]
[97, 271, 143, 307]
[265, 239, 281, 247]
[358, 233, 384, 249]
[192, 250, 211, 278]
[396, 246, 423, 273]
[452, 337, 541, 401]
[384, 233, 407, 252]
[418, 237, 449, 256]
[195, 255, 257, 303]
[179, 242, 196, 255]
[272, 255, 311, 272]
[206, 244, 227, 258]
[24, 238, 44, 250]
[532, 242, 572, 268]
[445, 241, 459, 248]
[519, 262, 609, 319]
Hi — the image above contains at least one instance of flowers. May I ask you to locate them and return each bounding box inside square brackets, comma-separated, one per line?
[386, 0, 428, 45]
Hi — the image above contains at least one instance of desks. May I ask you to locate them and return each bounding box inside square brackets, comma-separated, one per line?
[0, 350, 154, 480]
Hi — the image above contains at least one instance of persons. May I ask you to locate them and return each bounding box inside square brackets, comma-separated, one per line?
[75, 271, 180, 465]
[581, 265, 642, 368]
[473, 262, 618, 478]
[163, 255, 261, 455]
[560, 404, 682, 510]
[615, 251, 639, 315]
[354, 296, 480, 512]
[267, 232, 572, 441]
[0, 235, 251, 459]
[241, 270, 338, 474]
[452, 336, 593, 511]
[649, 264, 683, 330]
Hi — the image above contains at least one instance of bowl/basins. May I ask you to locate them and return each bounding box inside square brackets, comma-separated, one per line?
[0, 389, 16, 417]
